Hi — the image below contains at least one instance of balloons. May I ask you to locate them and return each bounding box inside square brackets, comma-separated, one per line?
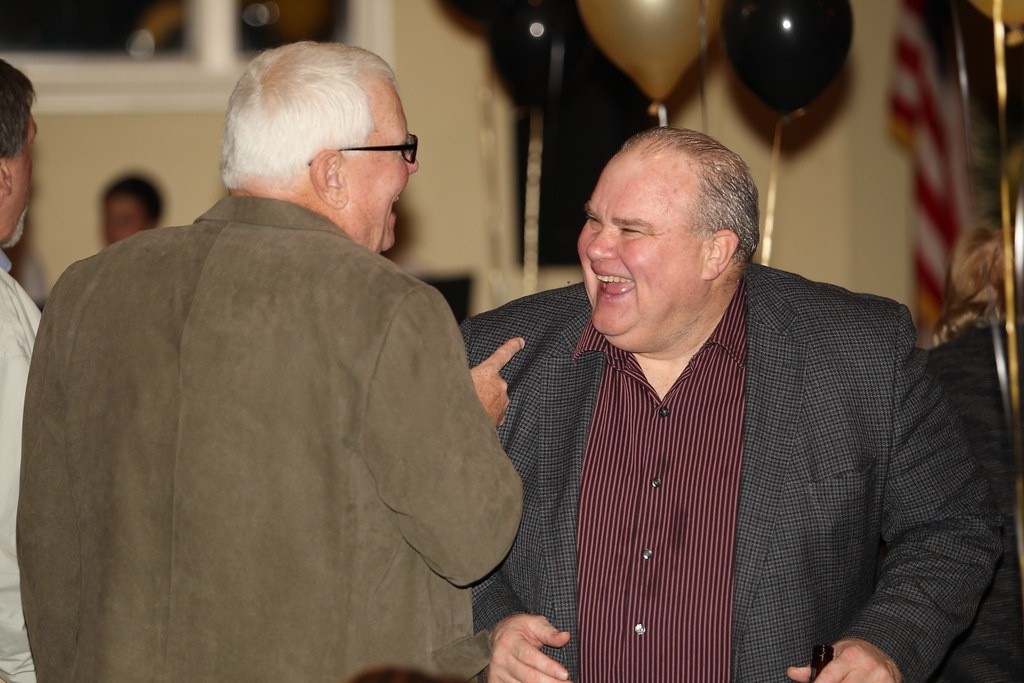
[579, 0, 720, 113]
[721, 1, 852, 112]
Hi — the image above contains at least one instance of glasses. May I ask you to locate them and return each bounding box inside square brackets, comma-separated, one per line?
[309, 132, 419, 166]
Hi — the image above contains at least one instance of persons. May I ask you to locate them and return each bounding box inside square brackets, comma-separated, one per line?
[12, 37, 527, 683]
[452, 126, 1001, 683]
[928, 142, 1024, 683]
[102, 171, 162, 244]
[0, 56, 42, 669]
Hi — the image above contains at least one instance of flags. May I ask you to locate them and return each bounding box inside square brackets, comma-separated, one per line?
[887, 11, 962, 344]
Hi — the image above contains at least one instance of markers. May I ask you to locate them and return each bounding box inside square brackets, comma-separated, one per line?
[809, 644, 835, 683]
